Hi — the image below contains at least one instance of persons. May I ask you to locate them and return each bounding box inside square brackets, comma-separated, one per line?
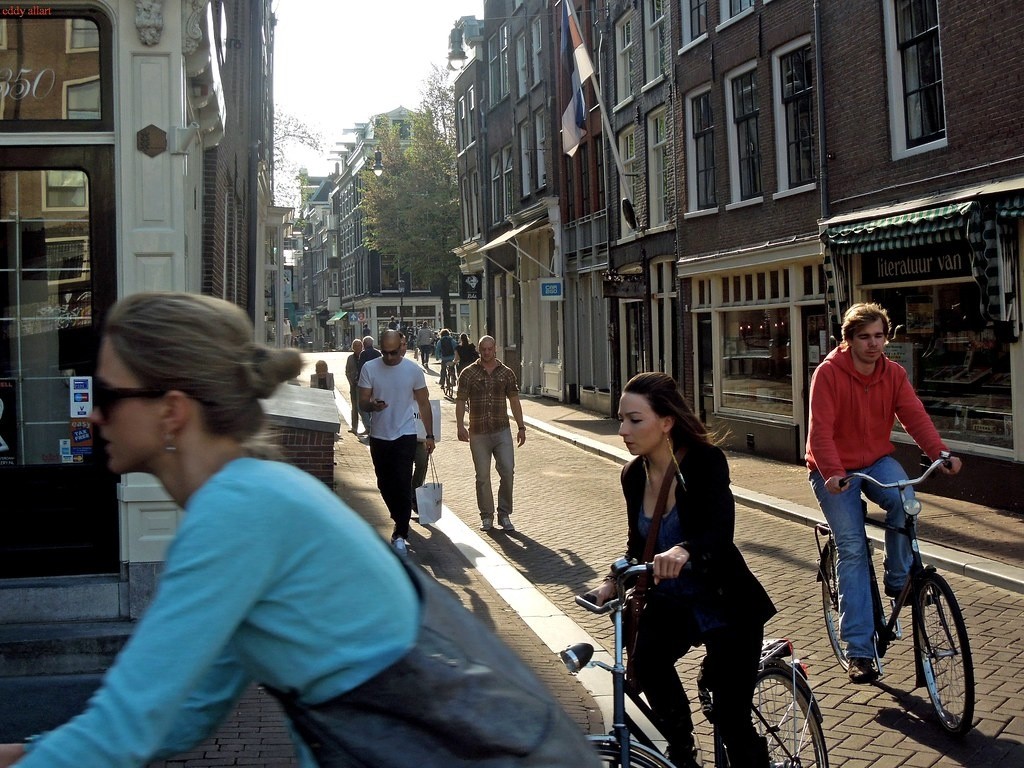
[346, 317, 479, 436]
[358, 329, 435, 557]
[456, 335, 526, 531]
[584, 372, 778, 768]
[804, 302, 962, 684]
[0, 292, 606, 768]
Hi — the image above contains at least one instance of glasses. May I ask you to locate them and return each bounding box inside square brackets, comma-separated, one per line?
[96, 388, 165, 421]
[382, 346, 399, 355]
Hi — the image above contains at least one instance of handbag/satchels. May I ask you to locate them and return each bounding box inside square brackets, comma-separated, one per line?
[610, 592, 647, 662]
[416, 448, 442, 524]
[258, 543, 604, 768]
[412, 400, 441, 442]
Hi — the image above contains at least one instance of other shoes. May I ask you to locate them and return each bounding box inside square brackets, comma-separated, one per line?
[885, 585, 930, 597]
[848, 657, 878, 683]
[480, 518, 493, 530]
[498, 517, 514, 530]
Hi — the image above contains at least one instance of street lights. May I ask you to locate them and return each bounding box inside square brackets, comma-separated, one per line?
[397, 278, 405, 331]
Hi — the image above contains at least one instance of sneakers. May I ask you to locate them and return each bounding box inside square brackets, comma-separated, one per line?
[394, 538, 407, 556]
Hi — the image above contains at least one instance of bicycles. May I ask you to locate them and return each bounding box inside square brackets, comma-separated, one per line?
[562, 562, 830, 768]
[815, 454, 975, 736]
[441, 363, 455, 398]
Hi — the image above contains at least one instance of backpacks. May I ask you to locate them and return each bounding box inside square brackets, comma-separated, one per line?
[440, 337, 454, 357]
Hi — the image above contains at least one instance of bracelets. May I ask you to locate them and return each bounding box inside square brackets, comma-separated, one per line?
[518, 426, 525, 430]
[426, 435, 434, 439]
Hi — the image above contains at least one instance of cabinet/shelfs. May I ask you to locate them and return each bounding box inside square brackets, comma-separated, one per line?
[893, 367, 1013, 449]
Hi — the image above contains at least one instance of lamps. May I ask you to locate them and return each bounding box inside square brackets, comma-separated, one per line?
[227, 38, 242, 49]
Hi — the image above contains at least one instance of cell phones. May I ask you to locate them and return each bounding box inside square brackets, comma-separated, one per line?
[377, 400, 385, 405]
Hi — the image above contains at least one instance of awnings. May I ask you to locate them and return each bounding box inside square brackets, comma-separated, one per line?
[470, 214, 555, 281]
[329, 311, 348, 321]
[819, 201, 988, 325]
[982, 196, 1024, 321]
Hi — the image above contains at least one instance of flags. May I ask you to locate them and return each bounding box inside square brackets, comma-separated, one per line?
[561, 0, 594, 158]
